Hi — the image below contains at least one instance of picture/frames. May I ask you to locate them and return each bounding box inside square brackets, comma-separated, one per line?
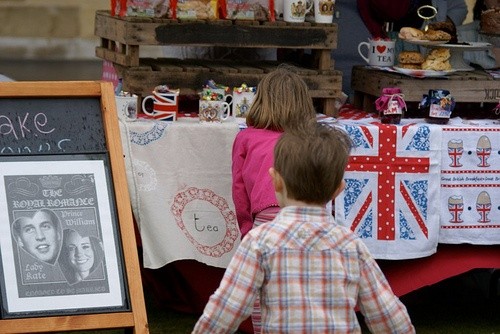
[0, 153, 132, 319]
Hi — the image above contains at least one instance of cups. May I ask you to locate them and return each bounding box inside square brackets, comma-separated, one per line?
[308, 0, 336, 23]
[358, 38, 396, 67]
[199, 88, 233, 122]
[233, 91, 257, 122]
[141, 91, 178, 121]
[115, 91, 138, 121]
[283, 0, 314, 22]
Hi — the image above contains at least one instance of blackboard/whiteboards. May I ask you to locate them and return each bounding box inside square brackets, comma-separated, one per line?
[0, 81, 148, 334]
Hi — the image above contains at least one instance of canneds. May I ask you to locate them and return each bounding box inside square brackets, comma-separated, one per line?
[375, 87, 405, 123]
[423, 89, 454, 124]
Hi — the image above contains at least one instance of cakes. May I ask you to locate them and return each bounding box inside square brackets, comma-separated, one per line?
[480, 0, 500, 35]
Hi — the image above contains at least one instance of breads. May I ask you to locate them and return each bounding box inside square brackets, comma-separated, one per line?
[398, 22, 453, 70]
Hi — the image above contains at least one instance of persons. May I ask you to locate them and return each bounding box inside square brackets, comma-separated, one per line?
[192, 117, 416, 334]
[232, 68, 317, 334]
[13, 210, 62, 265]
[58, 229, 104, 284]
[332, 0, 468, 104]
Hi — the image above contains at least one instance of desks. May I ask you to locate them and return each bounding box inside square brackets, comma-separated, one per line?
[120, 105, 500, 297]
